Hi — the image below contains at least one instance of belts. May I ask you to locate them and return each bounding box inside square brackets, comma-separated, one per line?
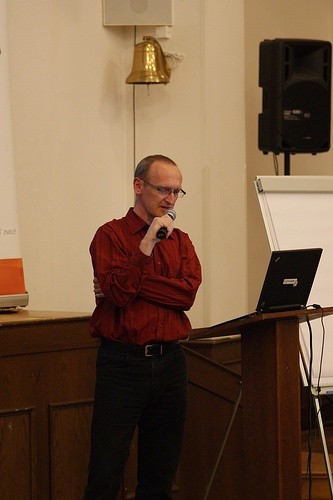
[102, 341, 179, 357]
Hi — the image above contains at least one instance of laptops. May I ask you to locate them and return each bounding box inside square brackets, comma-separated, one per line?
[210, 248, 323, 330]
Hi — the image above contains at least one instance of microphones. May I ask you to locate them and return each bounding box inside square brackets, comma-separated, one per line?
[156, 209, 176, 239]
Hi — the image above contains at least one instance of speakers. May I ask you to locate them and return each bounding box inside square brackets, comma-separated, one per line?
[257, 38, 332, 155]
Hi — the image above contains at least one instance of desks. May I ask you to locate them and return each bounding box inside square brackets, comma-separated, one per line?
[0, 309, 139, 500]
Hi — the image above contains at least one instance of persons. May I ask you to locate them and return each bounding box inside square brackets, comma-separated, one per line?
[89, 153, 202, 500]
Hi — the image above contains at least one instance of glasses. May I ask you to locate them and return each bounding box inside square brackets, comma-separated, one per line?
[143, 179, 186, 199]
